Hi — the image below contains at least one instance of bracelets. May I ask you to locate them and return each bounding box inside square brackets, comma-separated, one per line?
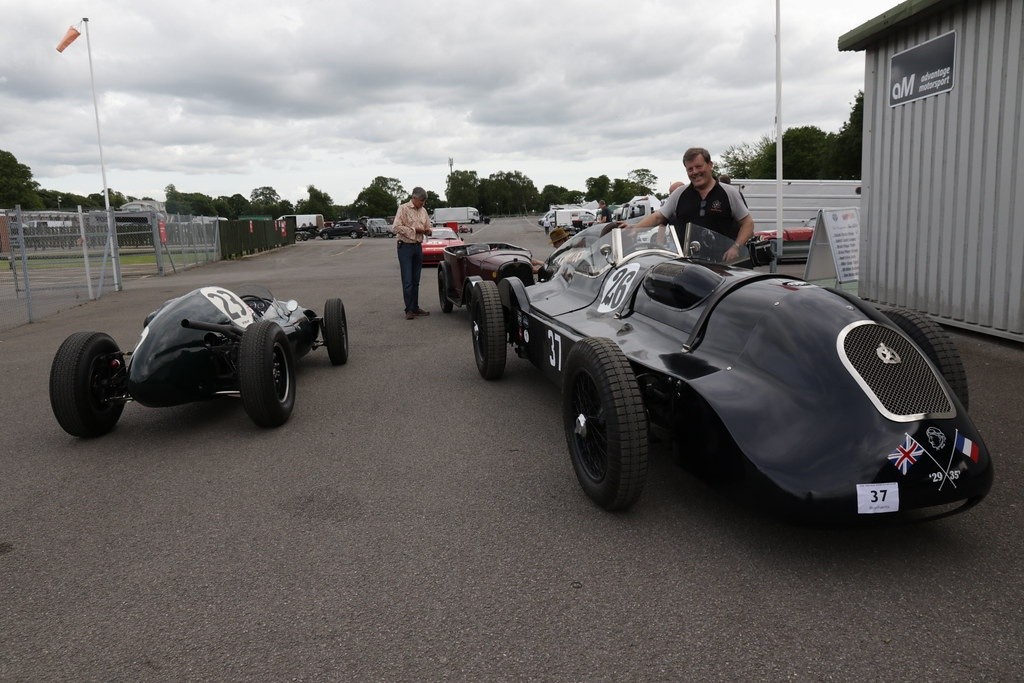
[734, 243, 743, 250]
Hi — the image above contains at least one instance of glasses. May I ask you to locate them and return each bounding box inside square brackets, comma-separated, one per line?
[699, 200, 707, 217]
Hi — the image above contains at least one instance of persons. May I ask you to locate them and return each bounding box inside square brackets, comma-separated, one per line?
[596, 200, 612, 224]
[392, 187, 433, 319]
[655, 175, 748, 249]
[544, 217, 551, 235]
[617, 148, 761, 272]
[531, 227, 569, 274]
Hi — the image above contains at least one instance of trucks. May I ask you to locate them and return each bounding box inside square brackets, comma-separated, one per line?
[435, 207, 479, 225]
[555, 210, 597, 229]
[276, 214, 324, 231]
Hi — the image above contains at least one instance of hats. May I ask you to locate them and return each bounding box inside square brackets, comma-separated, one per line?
[549, 227, 570, 244]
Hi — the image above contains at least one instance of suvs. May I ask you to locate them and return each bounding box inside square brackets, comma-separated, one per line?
[322, 220, 366, 239]
[366, 218, 387, 236]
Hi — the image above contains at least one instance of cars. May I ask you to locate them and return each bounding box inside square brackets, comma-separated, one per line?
[419, 227, 464, 265]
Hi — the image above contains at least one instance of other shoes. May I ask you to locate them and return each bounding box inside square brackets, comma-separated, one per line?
[406, 308, 415, 320]
[413, 307, 430, 317]
